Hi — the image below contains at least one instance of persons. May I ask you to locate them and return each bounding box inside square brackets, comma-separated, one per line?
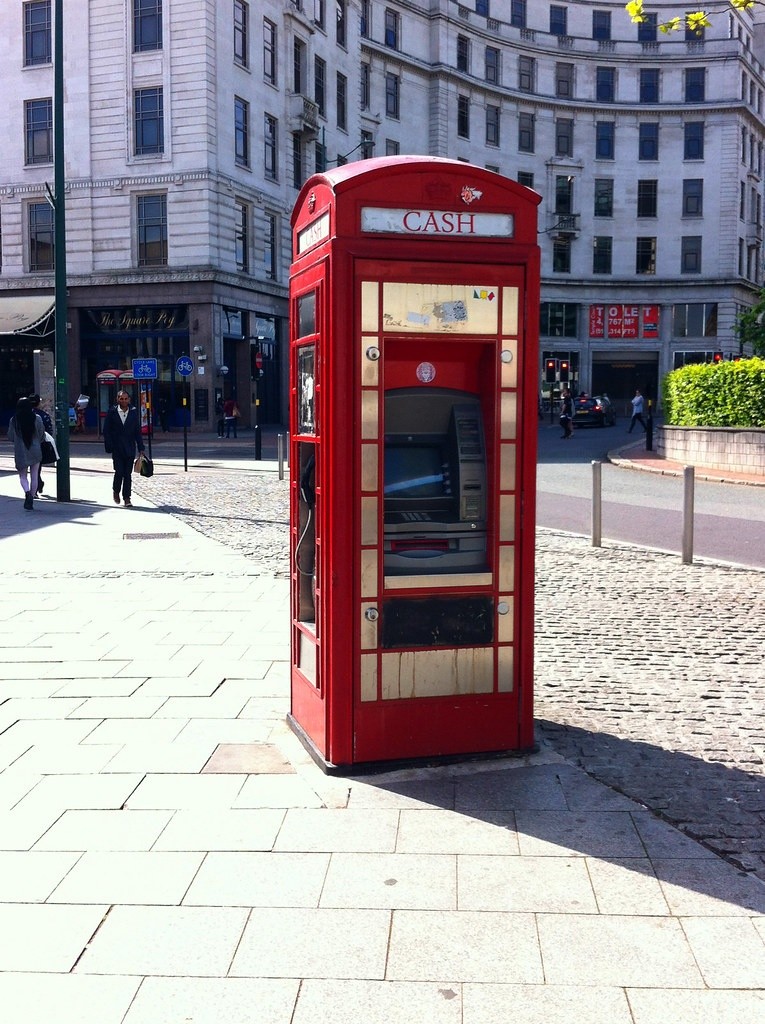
[69, 397, 88, 435]
[7, 398, 45, 511]
[625, 390, 648, 434]
[559, 387, 576, 439]
[31, 395, 54, 498]
[215, 394, 240, 439]
[103, 390, 145, 507]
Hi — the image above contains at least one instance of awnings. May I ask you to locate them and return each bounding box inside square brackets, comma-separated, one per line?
[0, 295, 56, 338]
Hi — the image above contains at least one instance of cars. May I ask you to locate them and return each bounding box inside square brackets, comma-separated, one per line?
[573, 397, 618, 427]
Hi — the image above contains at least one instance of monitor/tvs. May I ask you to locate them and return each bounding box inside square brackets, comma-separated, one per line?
[380, 430, 454, 512]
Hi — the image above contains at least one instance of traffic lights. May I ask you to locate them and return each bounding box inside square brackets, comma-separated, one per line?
[559, 360, 569, 383]
[713, 351, 724, 364]
[733, 355, 741, 362]
[545, 358, 556, 384]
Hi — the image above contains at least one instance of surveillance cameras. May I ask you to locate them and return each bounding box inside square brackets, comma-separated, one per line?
[194, 345, 202, 353]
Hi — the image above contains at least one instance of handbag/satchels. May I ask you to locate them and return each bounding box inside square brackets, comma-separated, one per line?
[232, 402, 240, 418]
[133, 454, 153, 478]
[43, 431, 59, 460]
[40, 439, 56, 464]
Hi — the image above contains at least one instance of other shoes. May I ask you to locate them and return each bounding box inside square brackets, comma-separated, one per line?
[23, 492, 33, 509]
[217, 435, 225, 438]
[113, 491, 120, 504]
[36, 477, 43, 493]
[123, 497, 131, 507]
[224, 436, 228, 438]
[233, 436, 237, 438]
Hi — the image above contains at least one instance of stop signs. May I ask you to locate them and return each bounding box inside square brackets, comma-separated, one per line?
[255, 353, 263, 368]
